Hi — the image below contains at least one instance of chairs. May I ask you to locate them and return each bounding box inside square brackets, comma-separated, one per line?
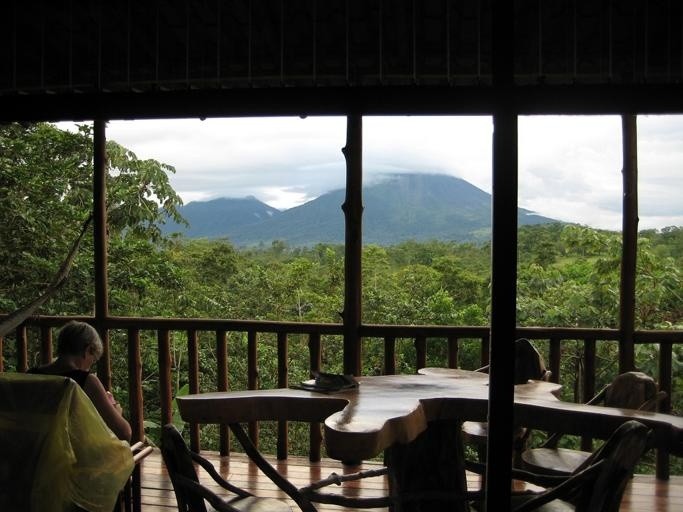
[463, 338, 668, 511]
[0, 375, 153, 510]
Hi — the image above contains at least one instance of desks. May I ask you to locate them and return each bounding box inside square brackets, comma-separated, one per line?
[176, 366, 683, 512]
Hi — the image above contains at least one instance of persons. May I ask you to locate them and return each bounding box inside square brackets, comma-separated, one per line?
[25, 319, 133, 512]
